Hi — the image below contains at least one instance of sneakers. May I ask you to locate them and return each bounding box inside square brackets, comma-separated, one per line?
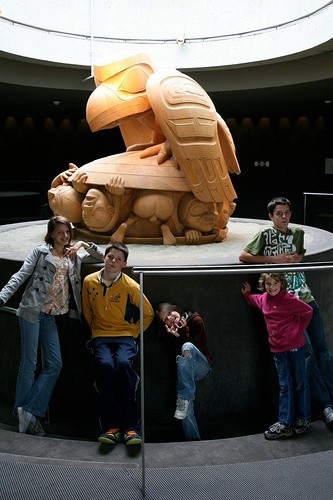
[124, 431, 143, 445]
[17, 407, 33, 433]
[321, 407, 333, 422]
[294, 418, 311, 433]
[26, 416, 45, 436]
[98, 428, 123, 444]
[264, 422, 293, 440]
[174, 398, 190, 419]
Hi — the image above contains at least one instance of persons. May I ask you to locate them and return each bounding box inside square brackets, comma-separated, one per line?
[0, 215, 104, 435]
[239, 197, 333, 425]
[156, 303, 212, 441]
[241, 271, 313, 440]
[82, 243, 154, 447]
